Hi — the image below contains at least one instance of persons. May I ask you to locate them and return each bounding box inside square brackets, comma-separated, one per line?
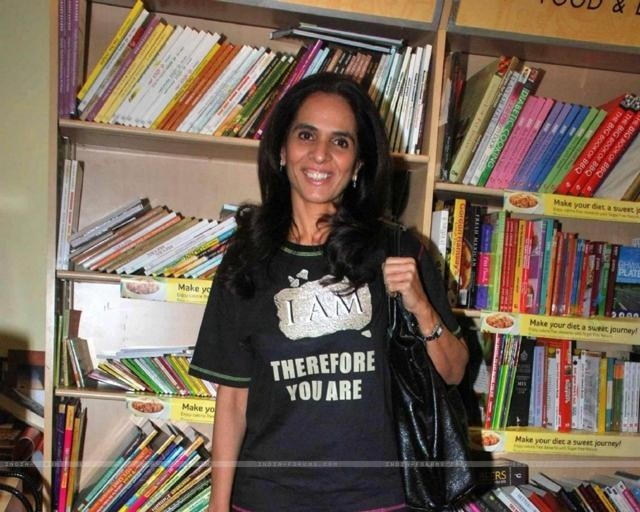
[187, 72, 471, 512]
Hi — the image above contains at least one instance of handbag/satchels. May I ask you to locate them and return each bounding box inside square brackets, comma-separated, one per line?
[382, 218, 491, 512]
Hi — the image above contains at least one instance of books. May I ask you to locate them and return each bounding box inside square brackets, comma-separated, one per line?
[58, 1, 433, 156]
[58, 159, 241, 281]
[454, 458, 640, 512]
[431, 198, 640, 433]
[2, 349, 45, 512]
[434, 48, 640, 204]
[51, 310, 218, 512]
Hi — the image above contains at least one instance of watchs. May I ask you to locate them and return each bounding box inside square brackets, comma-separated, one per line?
[420, 318, 443, 341]
